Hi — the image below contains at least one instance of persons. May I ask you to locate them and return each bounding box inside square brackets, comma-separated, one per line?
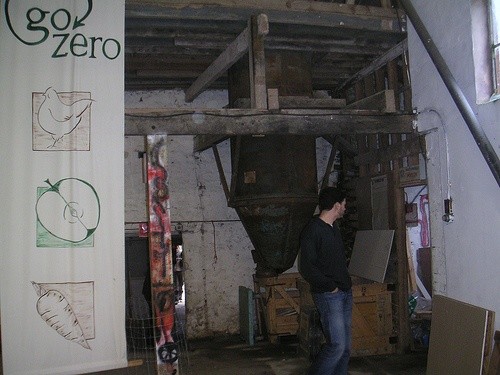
[171, 245, 184, 305]
[299, 185, 352, 375]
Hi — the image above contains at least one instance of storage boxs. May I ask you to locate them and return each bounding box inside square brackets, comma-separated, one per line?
[296, 276, 395, 357]
